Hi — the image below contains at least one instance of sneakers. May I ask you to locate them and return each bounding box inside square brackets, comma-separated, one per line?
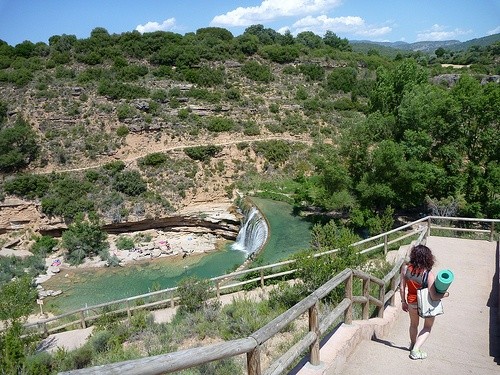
[409, 344, 414, 350]
[410, 350, 428, 359]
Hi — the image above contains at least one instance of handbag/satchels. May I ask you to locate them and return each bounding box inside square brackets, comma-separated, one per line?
[416, 269, 444, 318]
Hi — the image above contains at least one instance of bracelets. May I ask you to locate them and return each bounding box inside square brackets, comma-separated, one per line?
[401, 299, 406, 303]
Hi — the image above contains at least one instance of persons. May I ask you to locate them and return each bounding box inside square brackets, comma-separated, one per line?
[399, 245, 450, 360]
